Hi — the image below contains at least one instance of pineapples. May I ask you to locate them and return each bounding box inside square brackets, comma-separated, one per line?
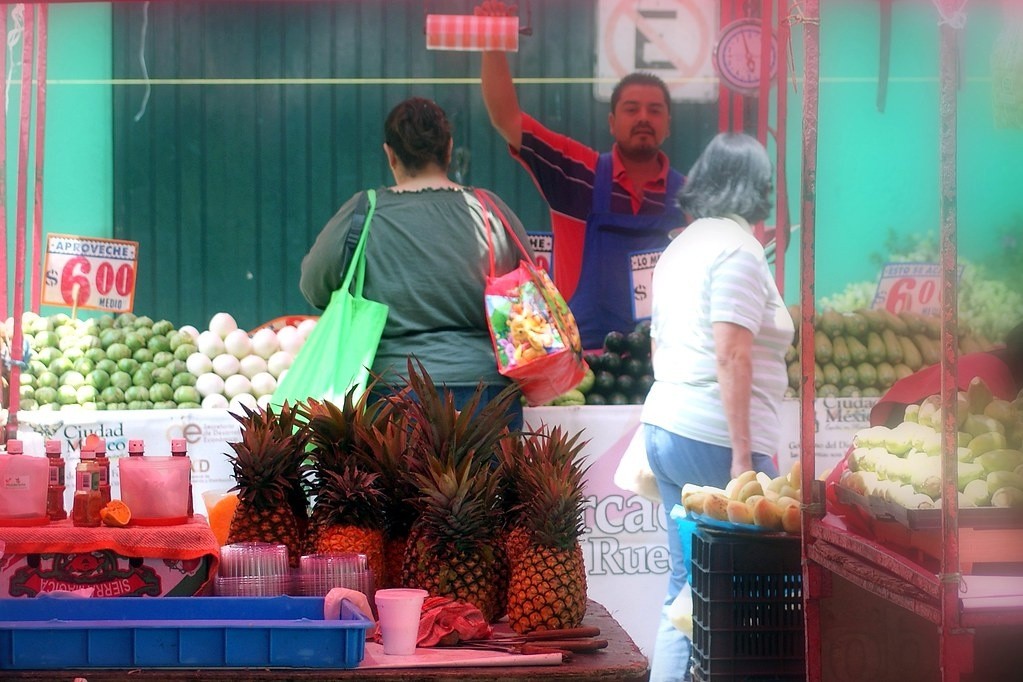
[230, 352, 587, 637]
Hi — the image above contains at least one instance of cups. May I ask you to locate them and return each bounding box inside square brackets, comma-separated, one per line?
[0, 456, 50, 527]
[118, 456, 190, 527]
[375, 588, 429, 656]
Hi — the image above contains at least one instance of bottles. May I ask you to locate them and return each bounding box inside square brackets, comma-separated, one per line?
[95, 440, 111, 512]
[171, 439, 194, 518]
[6, 439, 23, 455]
[44, 439, 67, 520]
[128, 439, 144, 457]
[73, 433, 101, 527]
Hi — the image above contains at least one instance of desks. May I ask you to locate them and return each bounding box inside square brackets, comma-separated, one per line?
[0, 599, 650, 682]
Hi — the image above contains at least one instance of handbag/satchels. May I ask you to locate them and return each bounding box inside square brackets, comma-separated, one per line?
[614, 422, 661, 502]
[476, 188, 589, 406]
[271, 189, 391, 452]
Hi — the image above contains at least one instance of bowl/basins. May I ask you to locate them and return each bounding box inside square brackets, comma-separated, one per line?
[214, 541, 372, 609]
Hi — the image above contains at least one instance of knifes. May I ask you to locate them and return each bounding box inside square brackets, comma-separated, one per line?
[417, 626, 608, 662]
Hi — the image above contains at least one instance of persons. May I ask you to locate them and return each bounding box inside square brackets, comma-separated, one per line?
[646, 132, 793, 682]
[299, 98, 537, 433]
[472, 0, 690, 351]
[868, 323, 1023, 429]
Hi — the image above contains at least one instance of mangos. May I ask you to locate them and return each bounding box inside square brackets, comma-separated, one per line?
[681, 462, 800, 534]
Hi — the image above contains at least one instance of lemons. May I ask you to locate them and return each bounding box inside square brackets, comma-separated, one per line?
[100, 499, 132, 526]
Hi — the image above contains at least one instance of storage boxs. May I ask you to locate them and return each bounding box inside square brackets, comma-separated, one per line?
[0, 595, 372, 668]
[686, 524, 805, 682]
